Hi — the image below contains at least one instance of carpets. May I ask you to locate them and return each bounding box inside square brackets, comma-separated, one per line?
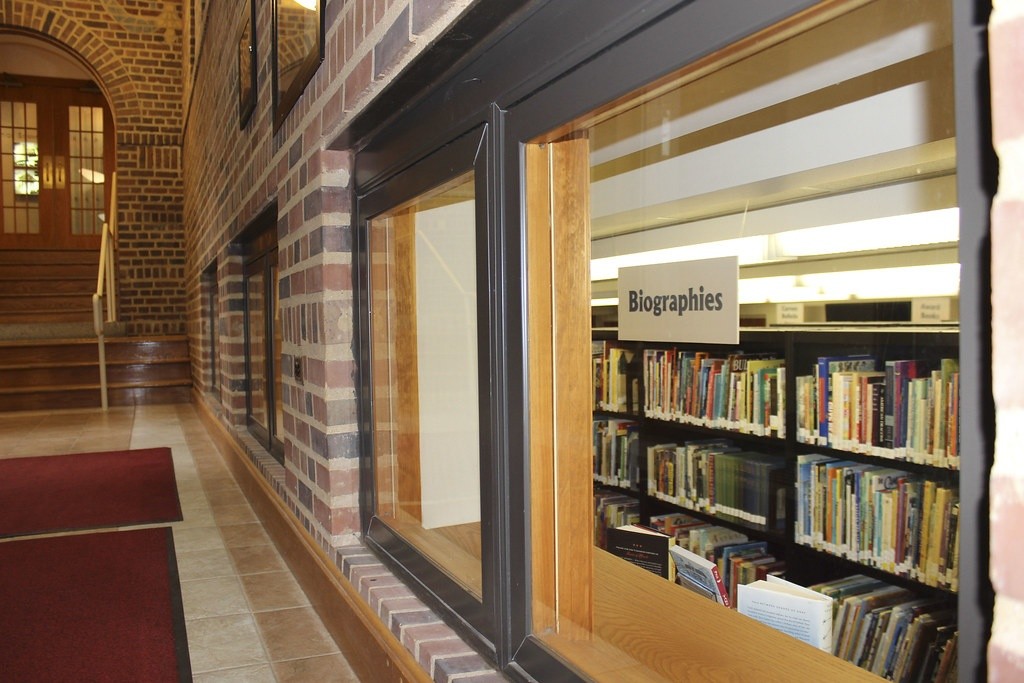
[0, 445, 184, 541]
[0, 527, 193, 683]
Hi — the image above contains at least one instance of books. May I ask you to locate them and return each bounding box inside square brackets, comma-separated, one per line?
[738, 574, 958, 683]
[593, 487, 785, 609]
[794, 454, 960, 592]
[590, 417, 791, 537]
[797, 353, 959, 458]
[589, 339, 786, 439]
